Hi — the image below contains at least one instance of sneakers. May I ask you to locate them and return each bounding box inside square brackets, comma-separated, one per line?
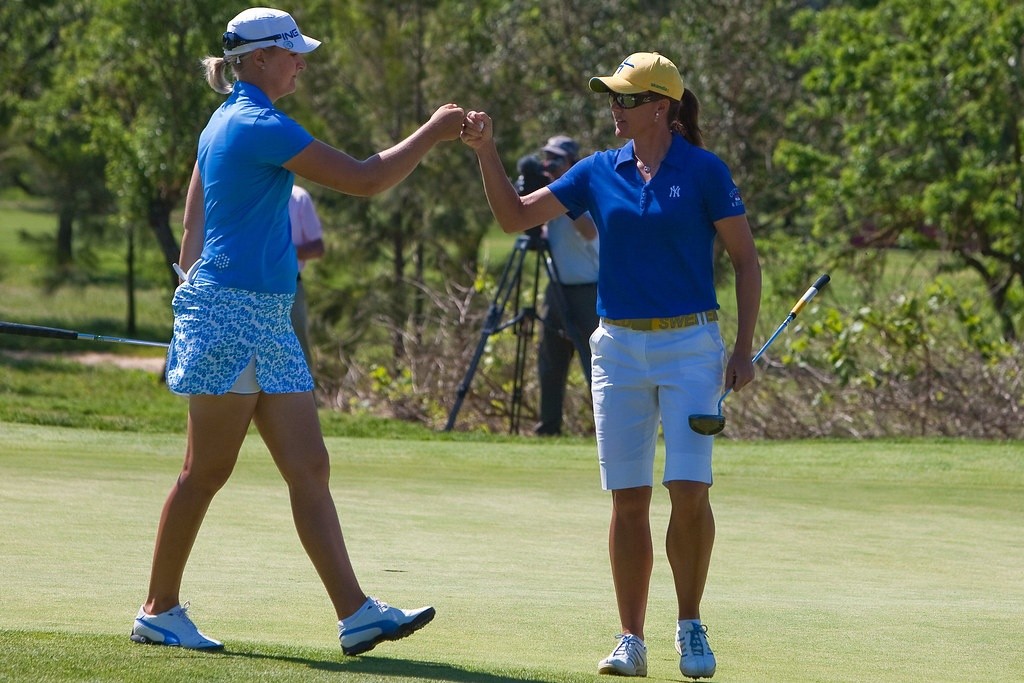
[337, 597, 433, 652]
[597, 633, 648, 675]
[130, 603, 223, 649]
[674, 619, 717, 679]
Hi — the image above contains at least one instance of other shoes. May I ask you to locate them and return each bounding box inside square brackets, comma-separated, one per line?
[528, 421, 560, 437]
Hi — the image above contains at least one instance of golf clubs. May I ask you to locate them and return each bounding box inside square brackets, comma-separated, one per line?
[0, 321, 170, 348]
[688, 272, 831, 435]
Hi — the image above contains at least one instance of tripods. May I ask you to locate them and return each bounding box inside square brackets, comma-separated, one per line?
[444, 227, 591, 437]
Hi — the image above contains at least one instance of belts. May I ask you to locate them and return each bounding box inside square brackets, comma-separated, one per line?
[603, 308, 718, 332]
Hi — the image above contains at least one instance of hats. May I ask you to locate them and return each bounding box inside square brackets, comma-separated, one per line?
[223, 6, 322, 58]
[589, 50, 684, 101]
[542, 136, 580, 160]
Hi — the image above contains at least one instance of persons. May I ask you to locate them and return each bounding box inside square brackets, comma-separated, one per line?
[533, 135, 600, 436]
[131, 8, 465, 656]
[289, 184, 325, 374]
[460, 52, 762, 680]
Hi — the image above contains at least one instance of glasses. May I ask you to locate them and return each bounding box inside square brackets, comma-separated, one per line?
[223, 32, 282, 51]
[607, 92, 665, 108]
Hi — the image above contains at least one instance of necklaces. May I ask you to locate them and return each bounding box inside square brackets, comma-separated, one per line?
[635, 154, 663, 172]
